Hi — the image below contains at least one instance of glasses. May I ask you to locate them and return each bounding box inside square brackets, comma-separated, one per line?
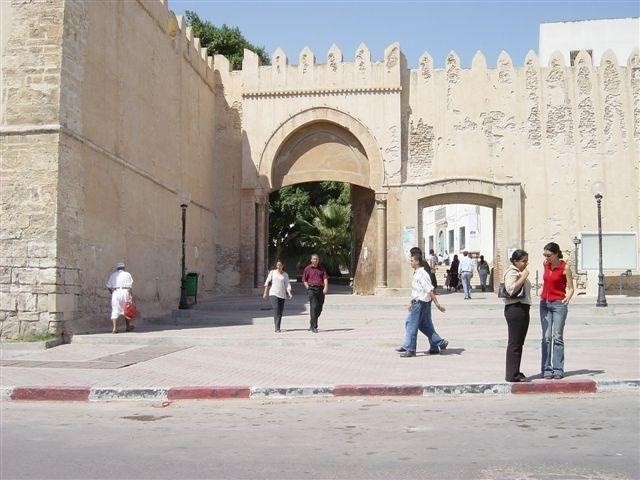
[542, 253, 556, 258]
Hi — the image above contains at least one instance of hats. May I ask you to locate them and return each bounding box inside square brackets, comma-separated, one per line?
[116, 262, 125, 268]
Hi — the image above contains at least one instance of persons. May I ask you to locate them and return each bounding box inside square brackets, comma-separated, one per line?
[540, 242, 574, 380]
[500, 250, 531, 382]
[389, 245, 490, 355]
[262, 259, 294, 332]
[302, 254, 328, 333]
[105, 261, 135, 333]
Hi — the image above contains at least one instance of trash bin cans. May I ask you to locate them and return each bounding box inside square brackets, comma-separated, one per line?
[185, 273, 198, 295]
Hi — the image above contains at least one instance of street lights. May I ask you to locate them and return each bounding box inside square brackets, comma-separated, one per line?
[176, 190, 193, 310]
[591, 180, 610, 308]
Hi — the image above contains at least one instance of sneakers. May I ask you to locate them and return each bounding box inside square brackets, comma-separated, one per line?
[519, 373, 564, 383]
[424, 340, 449, 356]
[395, 346, 417, 358]
[308, 326, 318, 333]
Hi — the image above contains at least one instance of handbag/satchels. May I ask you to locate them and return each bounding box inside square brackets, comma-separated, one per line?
[498, 268, 526, 299]
[123, 299, 137, 320]
[268, 281, 273, 290]
